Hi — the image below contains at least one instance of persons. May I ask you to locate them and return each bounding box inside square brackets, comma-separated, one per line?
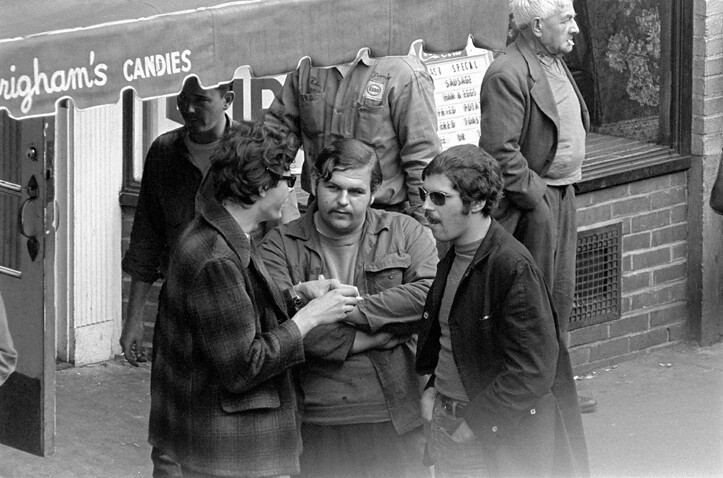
[147, 122, 360, 478]
[253, 137, 440, 478]
[120, 77, 298, 367]
[263, 49, 442, 227]
[415, 143, 590, 478]
[478, 0, 597, 412]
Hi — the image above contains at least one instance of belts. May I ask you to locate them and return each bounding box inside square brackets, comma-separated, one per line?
[441, 395, 469, 418]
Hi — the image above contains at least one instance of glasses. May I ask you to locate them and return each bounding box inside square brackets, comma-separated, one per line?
[417, 186, 464, 207]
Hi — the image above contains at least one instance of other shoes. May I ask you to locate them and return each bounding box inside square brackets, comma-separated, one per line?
[577, 396, 596, 412]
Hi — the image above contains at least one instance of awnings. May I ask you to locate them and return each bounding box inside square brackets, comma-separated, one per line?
[0, 0, 509, 121]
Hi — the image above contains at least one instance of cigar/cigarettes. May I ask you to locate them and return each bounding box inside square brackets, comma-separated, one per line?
[568, 39, 575, 45]
[356, 296, 364, 301]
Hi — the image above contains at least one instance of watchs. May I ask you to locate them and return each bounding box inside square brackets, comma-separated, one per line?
[289, 285, 305, 309]
